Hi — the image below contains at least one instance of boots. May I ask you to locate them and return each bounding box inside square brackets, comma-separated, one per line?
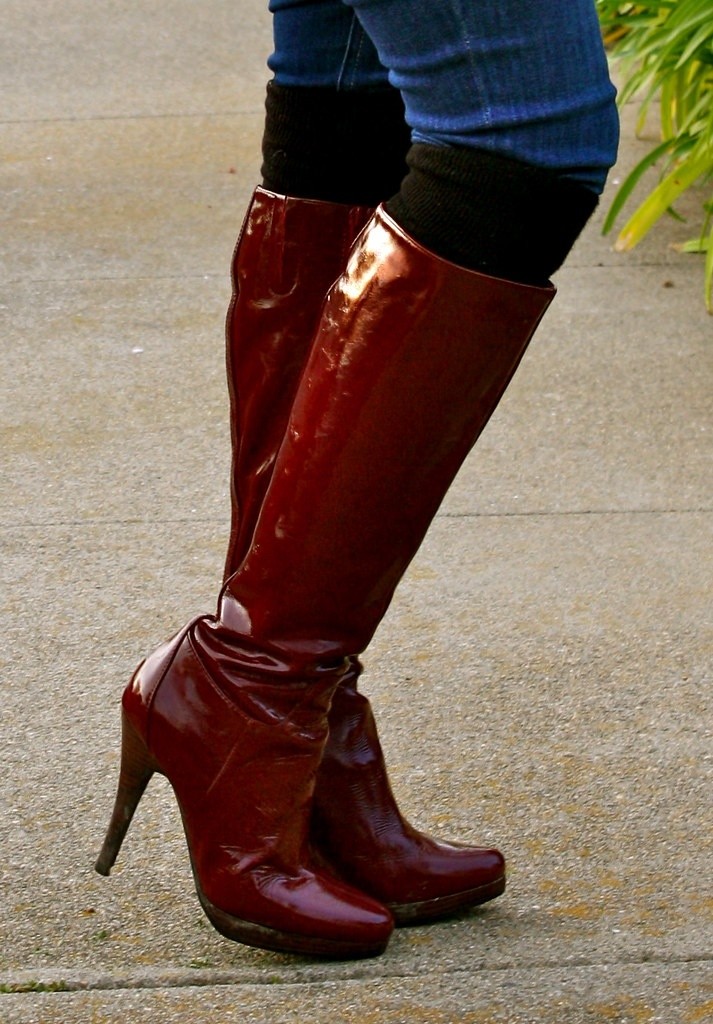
[96, 202, 561, 961]
[224, 185, 507, 924]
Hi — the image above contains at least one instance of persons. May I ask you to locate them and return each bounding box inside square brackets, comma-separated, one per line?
[96, 0, 620, 957]
